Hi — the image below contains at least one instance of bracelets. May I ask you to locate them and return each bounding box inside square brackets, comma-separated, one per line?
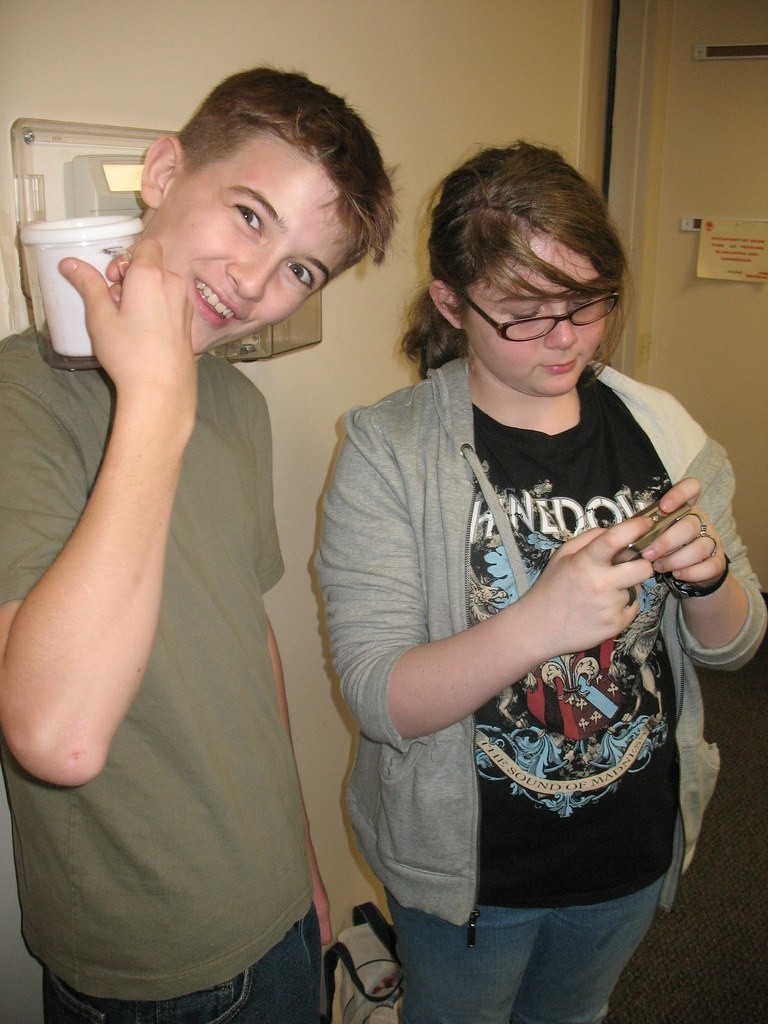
[662, 553, 737, 600]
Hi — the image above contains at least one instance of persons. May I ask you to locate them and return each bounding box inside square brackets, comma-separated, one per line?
[0, 66, 404, 1024]
[313, 139, 768, 1023]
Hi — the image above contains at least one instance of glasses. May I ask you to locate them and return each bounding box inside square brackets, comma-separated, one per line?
[454, 284, 618, 342]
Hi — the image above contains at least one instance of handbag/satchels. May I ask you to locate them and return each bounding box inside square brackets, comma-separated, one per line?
[324, 901, 404, 1024]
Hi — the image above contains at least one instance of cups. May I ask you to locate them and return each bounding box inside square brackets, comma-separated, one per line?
[20, 215, 144, 370]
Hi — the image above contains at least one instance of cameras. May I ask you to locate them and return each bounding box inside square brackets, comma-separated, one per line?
[611, 499, 692, 566]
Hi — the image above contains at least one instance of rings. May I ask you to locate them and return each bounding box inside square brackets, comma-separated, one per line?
[707, 532, 718, 554]
[683, 511, 707, 535]
